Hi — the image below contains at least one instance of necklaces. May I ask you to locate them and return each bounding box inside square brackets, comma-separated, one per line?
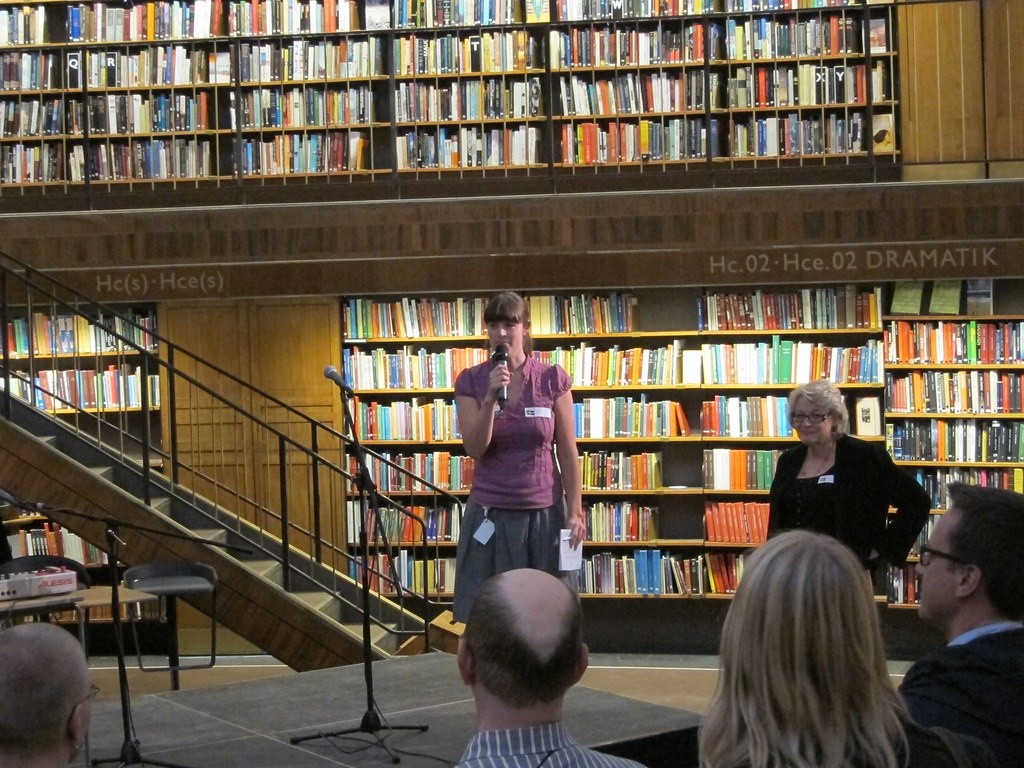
[802, 441, 835, 478]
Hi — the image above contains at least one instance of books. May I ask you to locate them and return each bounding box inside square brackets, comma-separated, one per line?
[342, 278, 1024, 597]
[20, 507, 168, 625]
[0, 310, 162, 412]
[0, 0, 897, 186]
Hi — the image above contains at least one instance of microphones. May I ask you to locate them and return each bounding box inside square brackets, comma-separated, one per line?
[0, 489, 31, 511]
[324, 366, 354, 398]
[495, 344, 508, 402]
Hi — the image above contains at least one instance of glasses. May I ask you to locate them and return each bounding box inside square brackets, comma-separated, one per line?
[82, 685, 100, 703]
[792, 411, 833, 423]
[920, 543, 967, 566]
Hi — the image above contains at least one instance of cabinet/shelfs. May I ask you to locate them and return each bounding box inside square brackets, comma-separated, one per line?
[340, 287, 881, 596]
[883, 315, 1024, 611]
[0, 306, 164, 626]
[0, 0, 894, 175]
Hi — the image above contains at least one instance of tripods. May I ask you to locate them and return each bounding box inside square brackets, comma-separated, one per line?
[22, 502, 253, 768]
[290, 389, 430, 763]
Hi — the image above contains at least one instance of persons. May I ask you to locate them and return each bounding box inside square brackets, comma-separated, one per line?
[766, 379, 931, 582]
[0, 623, 99, 768]
[899, 481, 1024, 768]
[455, 569, 648, 768]
[697, 532, 996, 768]
[453, 291, 588, 624]
